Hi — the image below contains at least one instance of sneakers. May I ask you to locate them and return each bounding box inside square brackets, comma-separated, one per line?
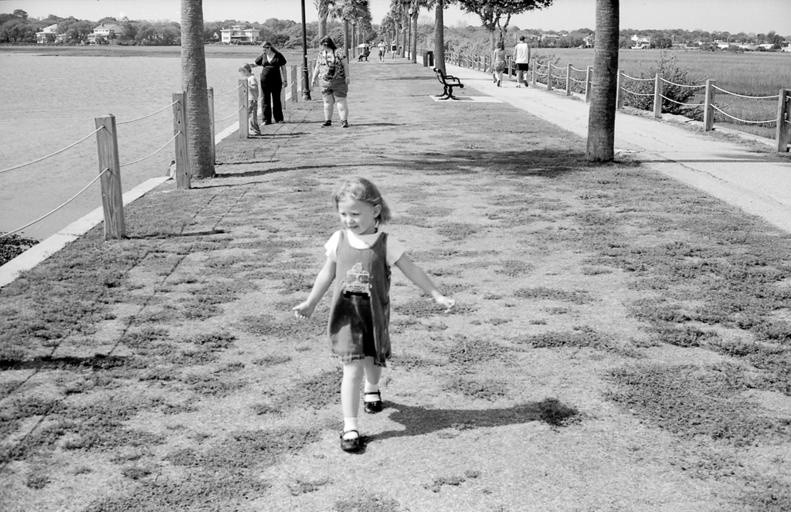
[322, 120, 348, 128]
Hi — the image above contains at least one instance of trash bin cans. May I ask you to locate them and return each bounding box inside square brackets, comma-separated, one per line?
[423, 51, 433, 70]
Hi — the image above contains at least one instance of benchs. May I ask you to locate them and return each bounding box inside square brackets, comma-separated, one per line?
[359, 55, 368, 61]
[433, 67, 464, 100]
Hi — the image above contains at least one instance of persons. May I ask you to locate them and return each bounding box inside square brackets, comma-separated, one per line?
[491, 41, 506, 88]
[310, 36, 351, 128]
[358, 39, 399, 63]
[288, 173, 457, 454]
[238, 63, 261, 136]
[251, 40, 288, 126]
[512, 36, 530, 87]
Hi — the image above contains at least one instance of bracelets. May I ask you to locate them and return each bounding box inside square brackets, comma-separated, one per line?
[283, 81, 287, 82]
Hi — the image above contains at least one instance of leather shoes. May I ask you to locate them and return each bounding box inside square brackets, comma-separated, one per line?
[364, 390, 381, 413]
[341, 430, 360, 451]
